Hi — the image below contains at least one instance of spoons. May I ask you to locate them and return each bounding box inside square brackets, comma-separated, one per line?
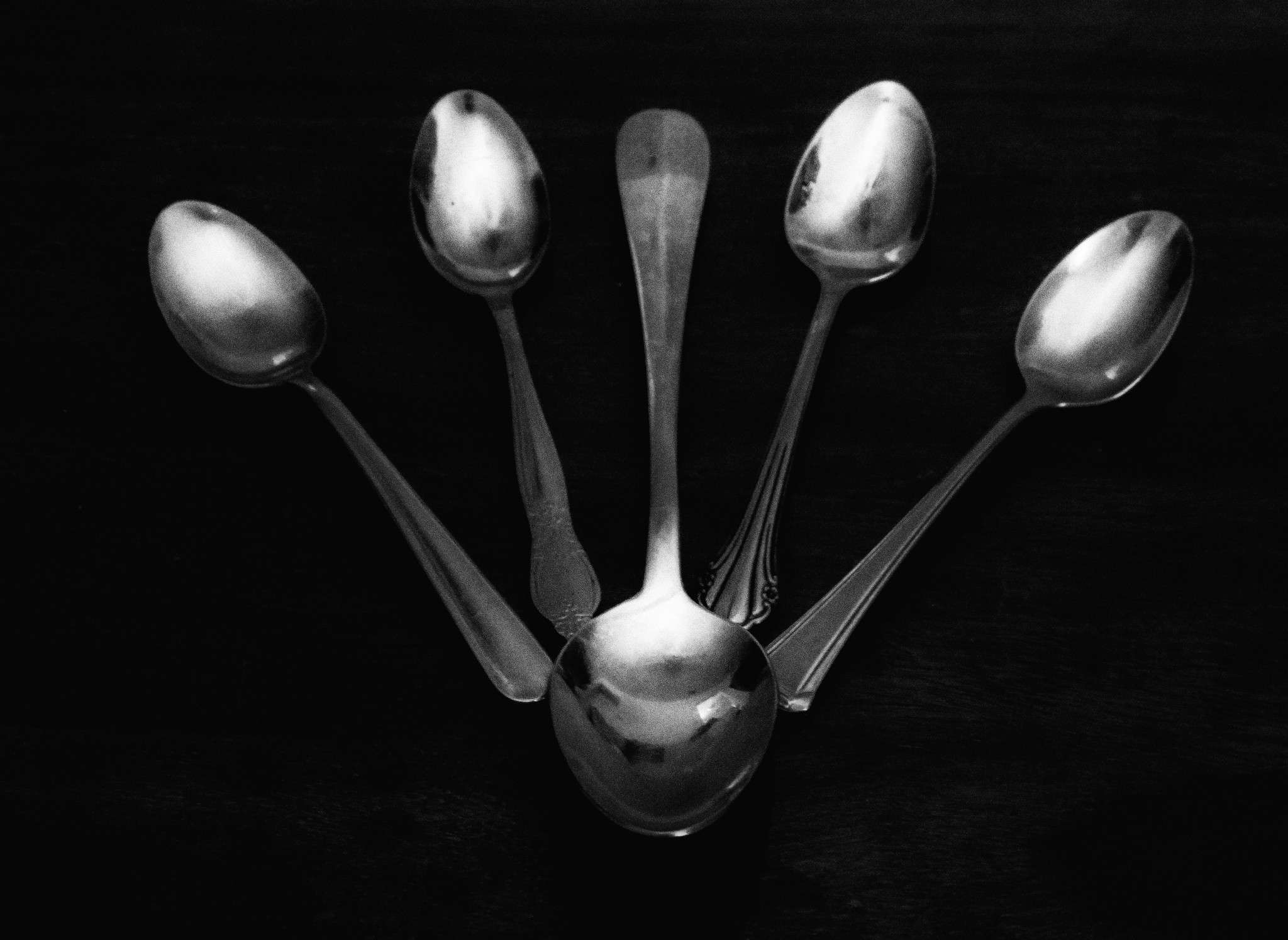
[146, 200, 552, 700]
[701, 78, 939, 625]
[409, 89, 600, 638]
[549, 105, 781, 842]
[762, 211, 1197, 713]
